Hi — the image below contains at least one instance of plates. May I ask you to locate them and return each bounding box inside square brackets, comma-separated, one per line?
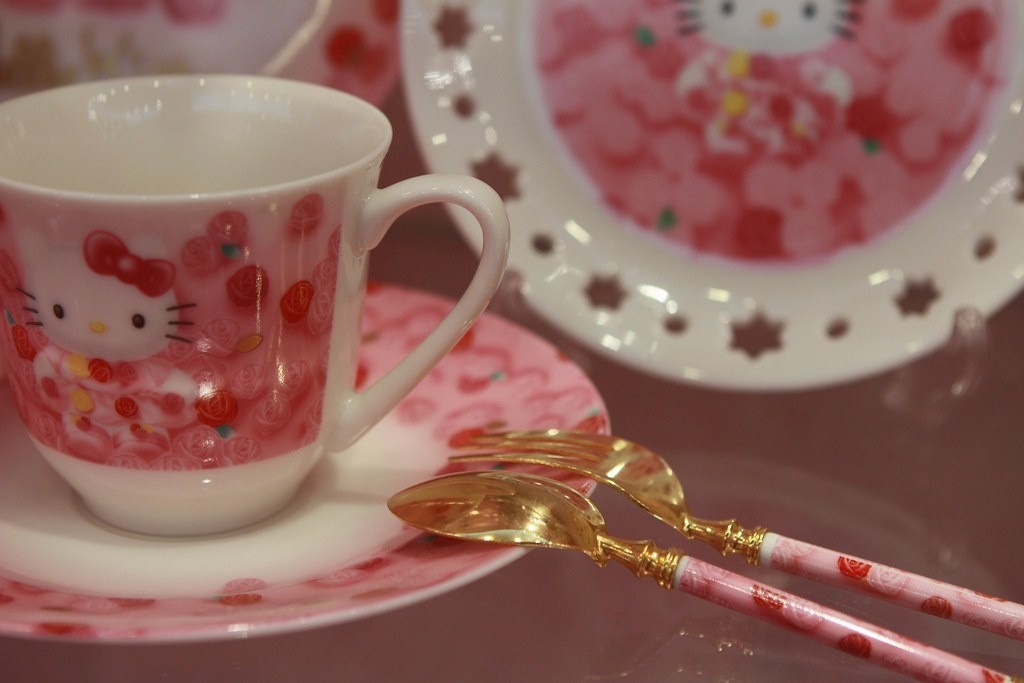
[393, 0, 1024, 393]
[0, 282, 607, 646]
[285, 1, 397, 111]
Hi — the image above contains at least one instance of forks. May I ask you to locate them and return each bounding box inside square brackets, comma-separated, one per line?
[450, 430, 1024, 641]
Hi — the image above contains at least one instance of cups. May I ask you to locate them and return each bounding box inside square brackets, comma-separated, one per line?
[1, 0, 329, 114]
[0, 74, 500, 537]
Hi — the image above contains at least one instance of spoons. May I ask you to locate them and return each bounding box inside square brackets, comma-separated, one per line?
[387, 468, 1024, 683]
